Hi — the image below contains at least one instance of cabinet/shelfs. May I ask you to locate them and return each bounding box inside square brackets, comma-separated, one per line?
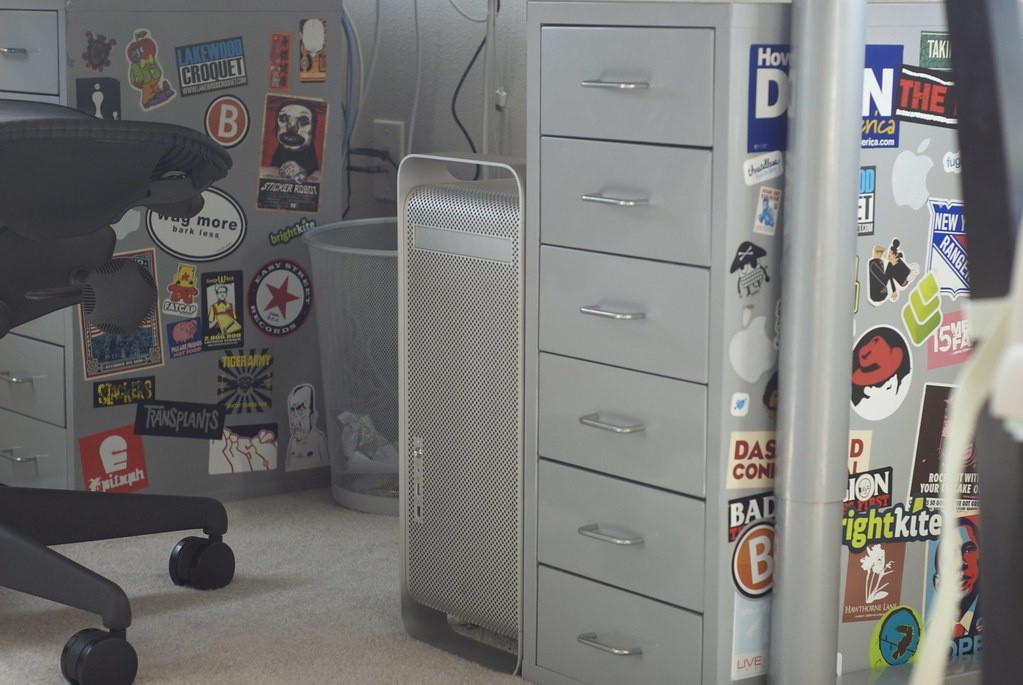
[519, 1, 981, 685]
[0, 0, 331, 501]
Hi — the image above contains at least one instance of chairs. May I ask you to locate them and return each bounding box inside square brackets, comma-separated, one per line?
[0, 97, 236, 685]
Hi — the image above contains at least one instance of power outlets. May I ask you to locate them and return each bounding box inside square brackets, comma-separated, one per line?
[373, 120, 406, 203]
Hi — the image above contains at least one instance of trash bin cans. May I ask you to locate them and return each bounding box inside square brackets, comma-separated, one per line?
[301, 215, 399, 513]
